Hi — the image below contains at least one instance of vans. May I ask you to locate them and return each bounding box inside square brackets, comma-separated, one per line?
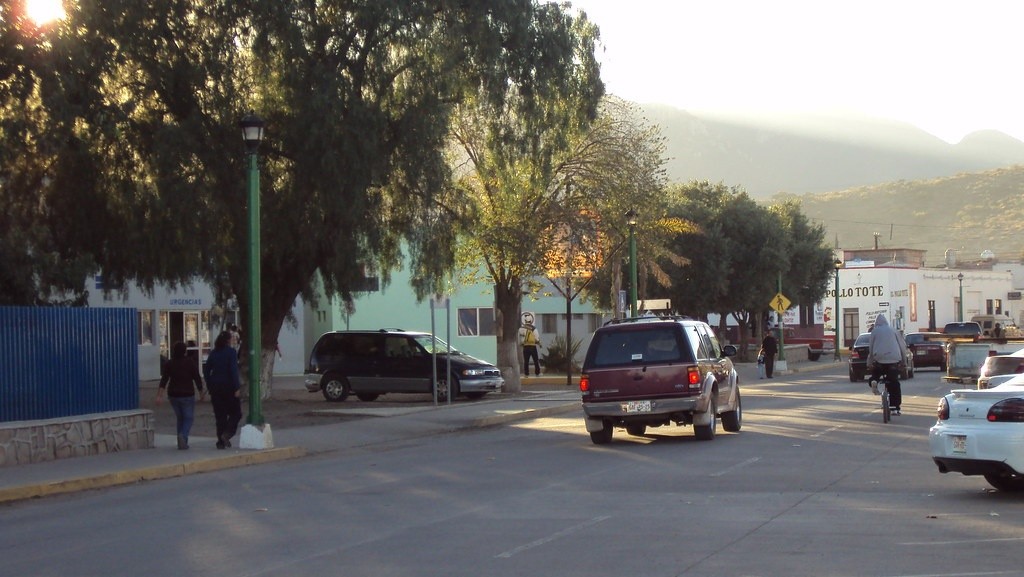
[944, 321, 983, 346]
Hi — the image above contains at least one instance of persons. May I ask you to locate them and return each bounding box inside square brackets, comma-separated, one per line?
[757, 330, 778, 379]
[868, 324, 874, 332]
[156, 342, 204, 449]
[204, 331, 242, 448]
[992, 323, 1004, 337]
[768, 316, 774, 330]
[869, 314, 907, 414]
[518, 316, 543, 377]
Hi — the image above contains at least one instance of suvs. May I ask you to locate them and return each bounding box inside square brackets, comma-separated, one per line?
[579, 314, 743, 447]
[303, 327, 506, 404]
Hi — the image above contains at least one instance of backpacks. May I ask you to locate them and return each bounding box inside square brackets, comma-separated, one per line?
[523, 326, 535, 344]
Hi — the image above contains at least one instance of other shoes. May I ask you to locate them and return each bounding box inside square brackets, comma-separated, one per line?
[891, 409, 897, 415]
[178, 444, 190, 450]
[767, 376, 773, 379]
[536, 372, 543, 377]
[221, 433, 232, 449]
[871, 381, 880, 395]
[524, 374, 529, 378]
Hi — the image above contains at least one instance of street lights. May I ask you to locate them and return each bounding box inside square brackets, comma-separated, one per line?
[234, 107, 274, 451]
[834, 258, 842, 361]
[956, 271, 964, 321]
[624, 200, 641, 323]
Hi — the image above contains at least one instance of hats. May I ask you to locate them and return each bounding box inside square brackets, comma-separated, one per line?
[525, 316, 532, 322]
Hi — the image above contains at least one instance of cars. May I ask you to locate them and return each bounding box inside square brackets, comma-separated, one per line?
[848, 331, 948, 382]
[929, 345, 1024, 492]
